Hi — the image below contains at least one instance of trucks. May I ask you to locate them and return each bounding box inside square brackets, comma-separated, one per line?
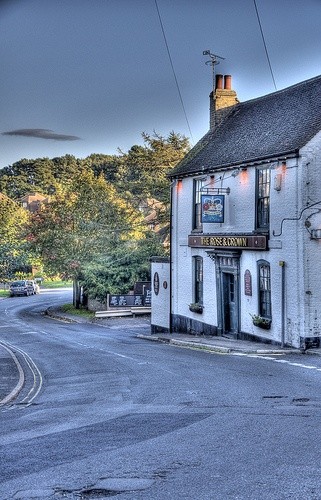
[9, 281, 34, 297]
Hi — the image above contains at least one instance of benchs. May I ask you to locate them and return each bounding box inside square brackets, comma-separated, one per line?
[131, 307, 152, 318]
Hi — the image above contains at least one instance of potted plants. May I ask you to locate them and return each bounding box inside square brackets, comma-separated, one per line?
[249, 313, 271, 330]
[188, 302, 204, 314]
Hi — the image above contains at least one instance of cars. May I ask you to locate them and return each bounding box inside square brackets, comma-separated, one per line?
[34, 280, 40, 295]
